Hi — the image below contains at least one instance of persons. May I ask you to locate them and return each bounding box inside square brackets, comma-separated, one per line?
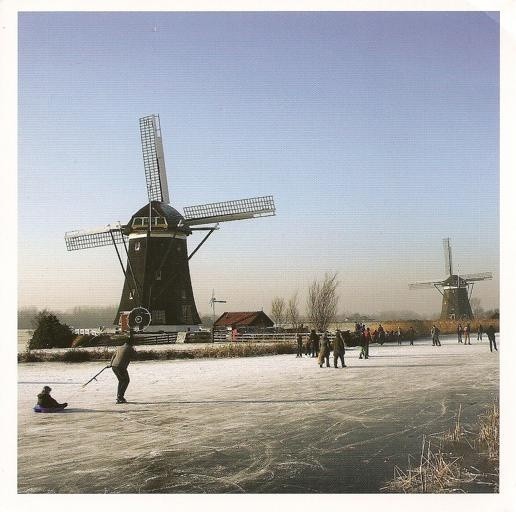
[485, 324, 497, 352]
[477, 324, 482, 340]
[405, 326, 417, 345]
[457, 324, 463, 343]
[36, 386, 68, 408]
[430, 325, 441, 346]
[395, 326, 405, 346]
[463, 326, 472, 345]
[107, 338, 139, 404]
[295, 321, 385, 368]
